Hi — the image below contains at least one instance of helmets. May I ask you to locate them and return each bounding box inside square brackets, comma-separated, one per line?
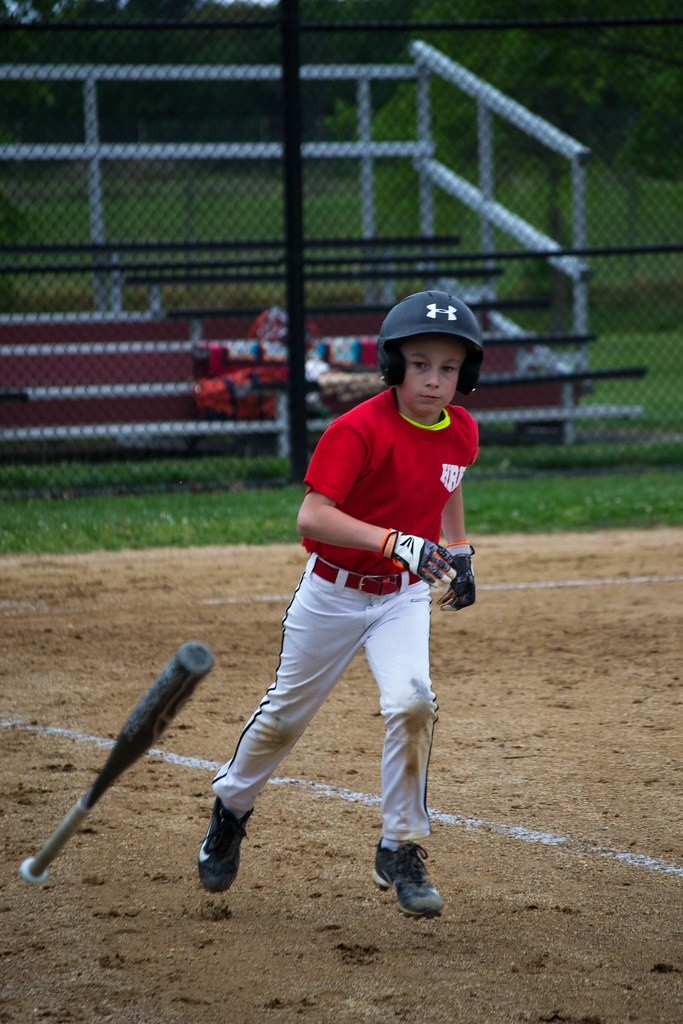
[377, 290, 484, 396]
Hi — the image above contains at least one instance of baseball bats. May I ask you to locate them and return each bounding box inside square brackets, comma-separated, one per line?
[16, 642, 213, 886]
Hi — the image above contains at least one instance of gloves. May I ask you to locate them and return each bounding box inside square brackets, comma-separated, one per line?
[381, 528, 457, 588]
[437, 542, 477, 612]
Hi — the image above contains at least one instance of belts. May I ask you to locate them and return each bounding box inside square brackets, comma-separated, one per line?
[311, 558, 421, 596]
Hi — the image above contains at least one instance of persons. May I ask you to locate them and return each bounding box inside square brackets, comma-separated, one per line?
[198, 290, 484, 916]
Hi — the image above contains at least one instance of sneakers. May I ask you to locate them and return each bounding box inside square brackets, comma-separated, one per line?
[198, 796, 254, 891]
[373, 837, 444, 915]
